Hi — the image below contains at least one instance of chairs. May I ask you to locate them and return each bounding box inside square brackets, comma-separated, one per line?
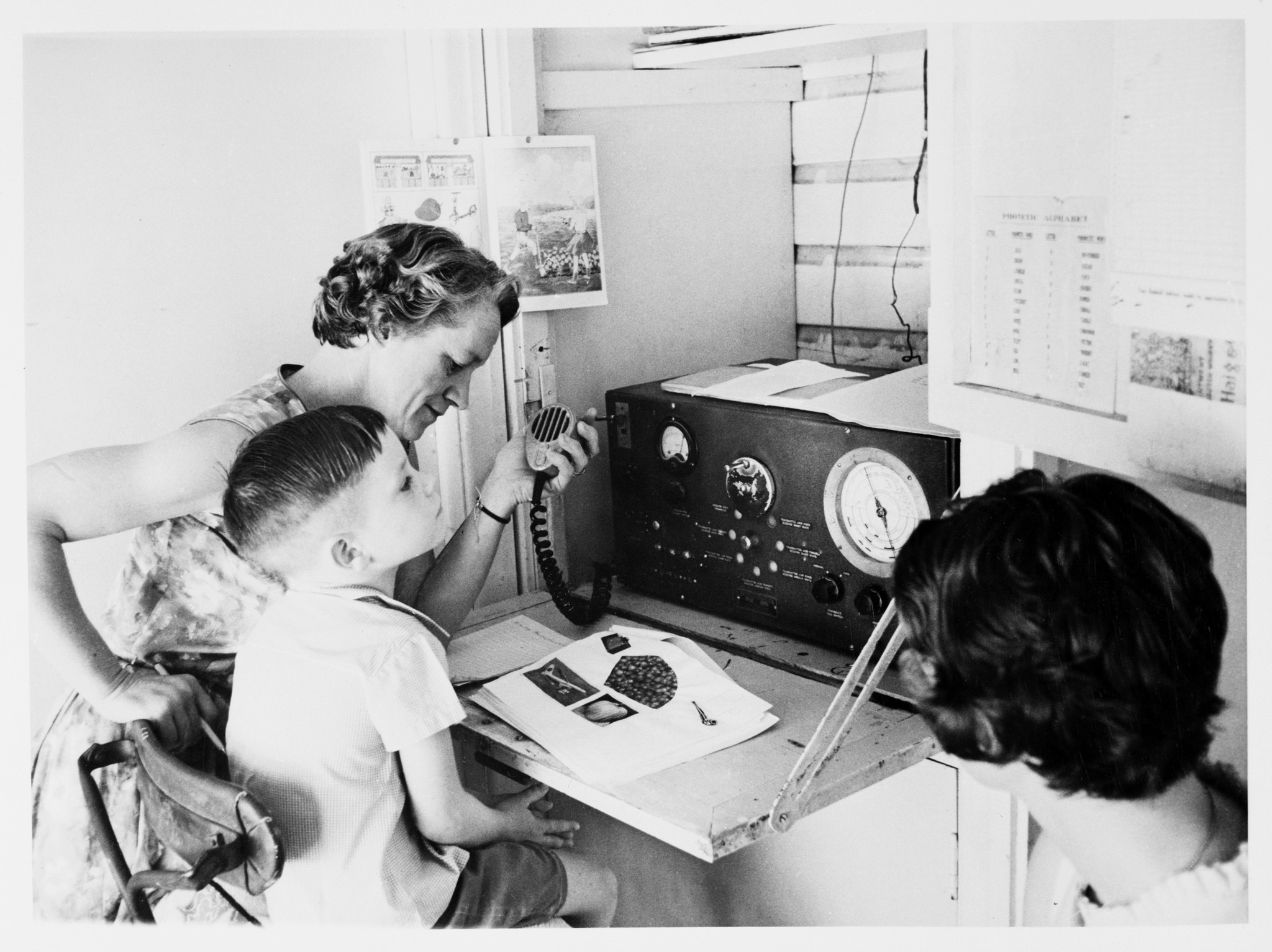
[78, 719, 287, 923]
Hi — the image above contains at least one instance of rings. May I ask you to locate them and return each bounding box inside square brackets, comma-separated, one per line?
[573, 467, 587, 479]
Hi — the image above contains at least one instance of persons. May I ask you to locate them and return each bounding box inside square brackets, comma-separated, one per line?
[887, 458, 1250, 925]
[219, 402, 621, 930]
[31, 217, 603, 929]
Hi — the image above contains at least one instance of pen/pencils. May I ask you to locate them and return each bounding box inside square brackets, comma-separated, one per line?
[154, 661, 227, 757]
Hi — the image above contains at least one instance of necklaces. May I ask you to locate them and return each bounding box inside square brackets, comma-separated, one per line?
[1178, 780, 1220, 876]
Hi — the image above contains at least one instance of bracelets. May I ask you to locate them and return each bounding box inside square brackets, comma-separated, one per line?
[471, 495, 512, 543]
